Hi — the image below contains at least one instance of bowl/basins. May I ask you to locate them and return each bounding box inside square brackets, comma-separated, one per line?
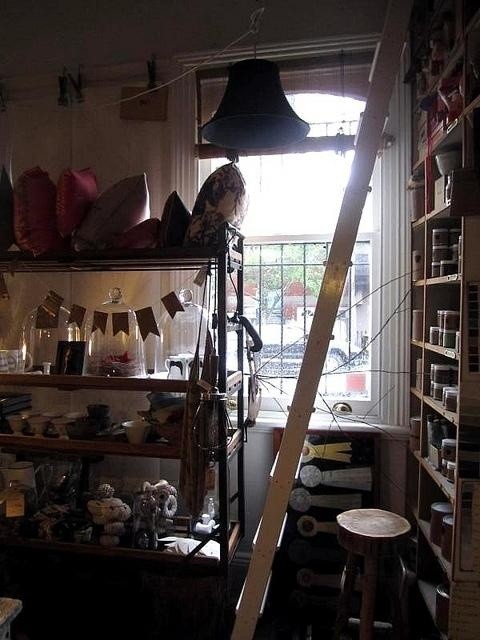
[434, 151, 462, 177]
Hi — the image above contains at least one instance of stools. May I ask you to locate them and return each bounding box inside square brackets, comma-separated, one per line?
[337, 507, 412, 638]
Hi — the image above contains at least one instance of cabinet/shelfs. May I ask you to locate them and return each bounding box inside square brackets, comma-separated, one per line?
[0, 222, 245, 565]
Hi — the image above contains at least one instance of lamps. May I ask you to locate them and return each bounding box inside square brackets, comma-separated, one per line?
[197, 5, 310, 150]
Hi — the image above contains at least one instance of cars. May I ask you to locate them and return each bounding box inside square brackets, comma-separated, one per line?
[252, 323, 367, 380]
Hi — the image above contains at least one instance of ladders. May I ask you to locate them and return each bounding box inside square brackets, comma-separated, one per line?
[230, 0, 412, 640]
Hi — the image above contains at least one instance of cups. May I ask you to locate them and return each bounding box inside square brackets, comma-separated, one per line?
[6, 400, 152, 447]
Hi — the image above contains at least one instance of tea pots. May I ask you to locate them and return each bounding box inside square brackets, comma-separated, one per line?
[1, 461, 53, 508]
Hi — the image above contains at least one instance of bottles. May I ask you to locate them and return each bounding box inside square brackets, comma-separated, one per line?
[18, 289, 218, 387]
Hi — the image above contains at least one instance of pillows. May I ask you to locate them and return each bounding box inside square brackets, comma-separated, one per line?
[0, 158, 247, 256]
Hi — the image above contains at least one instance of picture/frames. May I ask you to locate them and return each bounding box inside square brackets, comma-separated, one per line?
[271, 424, 384, 636]
